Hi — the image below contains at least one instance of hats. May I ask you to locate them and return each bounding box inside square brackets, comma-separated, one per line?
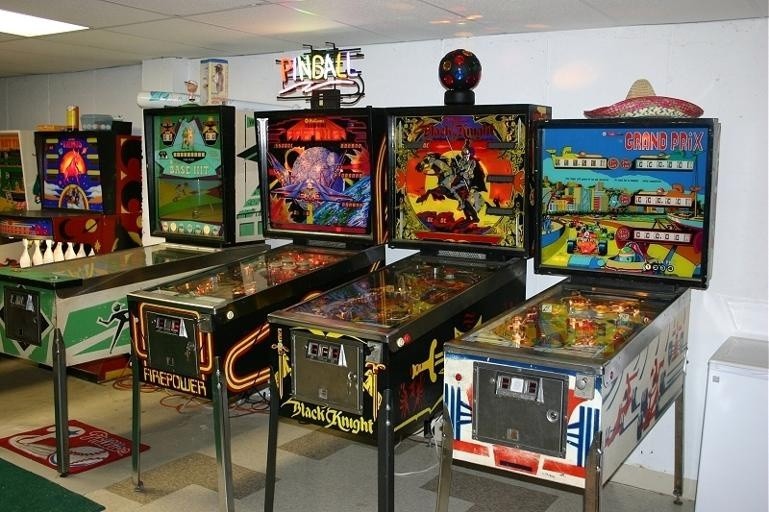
[583, 79, 704, 120]
